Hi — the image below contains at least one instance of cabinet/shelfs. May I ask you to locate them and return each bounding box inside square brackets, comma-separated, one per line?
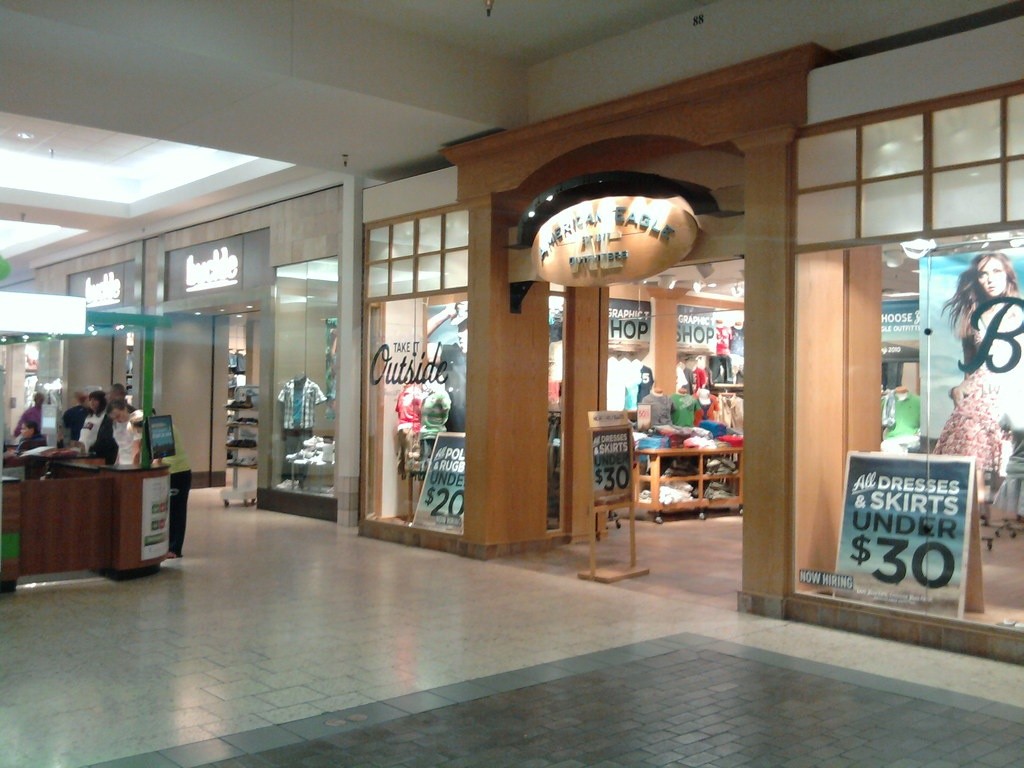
[629, 434, 744, 523]
[219, 384, 261, 507]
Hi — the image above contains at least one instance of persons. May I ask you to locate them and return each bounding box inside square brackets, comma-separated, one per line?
[879, 387, 923, 440]
[63, 390, 94, 445]
[642, 387, 676, 427]
[668, 387, 703, 427]
[106, 401, 144, 468]
[690, 387, 721, 429]
[3, 382, 48, 463]
[418, 380, 451, 480]
[87, 383, 138, 466]
[930, 256, 1024, 516]
[273, 371, 328, 492]
[395, 384, 423, 479]
[127, 407, 194, 558]
[75, 388, 109, 456]
